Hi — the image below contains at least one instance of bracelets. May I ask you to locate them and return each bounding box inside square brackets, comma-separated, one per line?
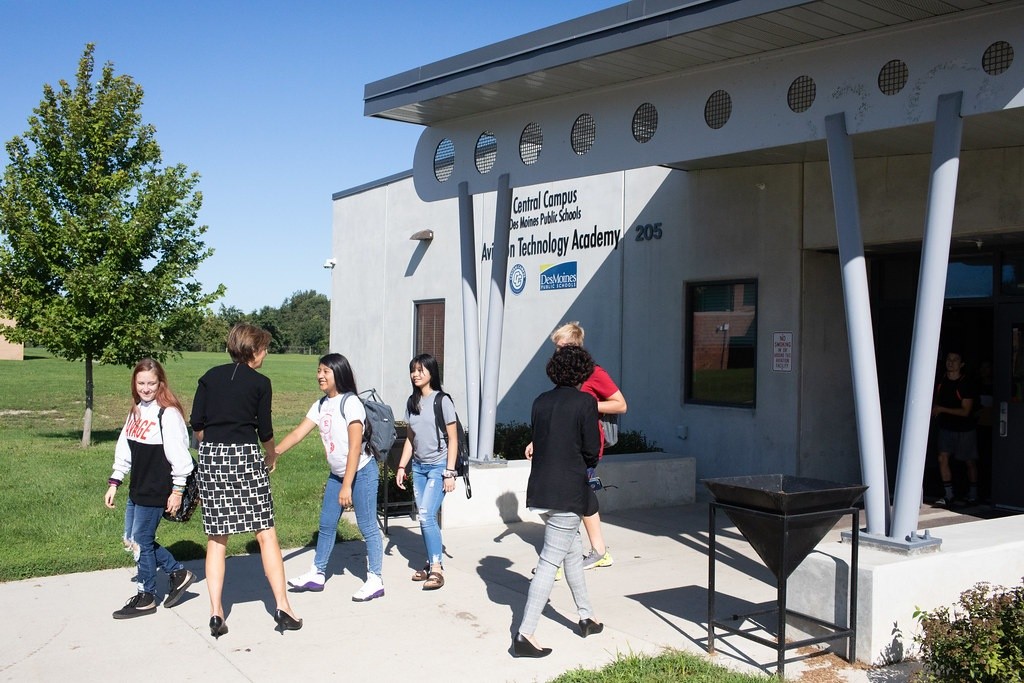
[442, 469, 458, 478]
[108, 483, 118, 487]
[398, 467, 405, 470]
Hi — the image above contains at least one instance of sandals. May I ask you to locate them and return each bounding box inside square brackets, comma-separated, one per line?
[423, 572, 444, 589]
[412, 565, 444, 581]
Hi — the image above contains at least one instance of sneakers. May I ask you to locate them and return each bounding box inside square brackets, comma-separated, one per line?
[112, 592, 156, 619]
[351, 571, 384, 602]
[163, 567, 197, 608]
[531, 567, 561, 581]
[582, 546, 614, 570]
[287, 565, 325, 592]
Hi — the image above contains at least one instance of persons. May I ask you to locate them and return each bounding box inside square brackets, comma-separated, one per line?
[265, 353, 385, 602]
[512, 344, 603, 658]
[189, 324, 303, 640]
[396, 353, 458, 590]
[524, 323, 628, 581]
[104, 359, 197, 620]
[930, 345, 994, 507]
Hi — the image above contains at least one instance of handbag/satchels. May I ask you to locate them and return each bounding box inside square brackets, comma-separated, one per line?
[157, 406, 198, 523]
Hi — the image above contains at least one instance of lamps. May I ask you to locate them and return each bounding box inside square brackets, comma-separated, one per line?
[409, 229, 433, 241]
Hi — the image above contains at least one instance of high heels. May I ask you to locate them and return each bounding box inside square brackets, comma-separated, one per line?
[513, 632, 552, 658]
[274, 609, 302, 635]
[209, 615, 228, 640]
[579, 618, 603, 638]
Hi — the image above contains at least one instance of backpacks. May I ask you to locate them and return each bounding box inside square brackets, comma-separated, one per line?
[407, 391, 471, 499]
[318, 388, 397, 463]
[575, 364, 618, 448]
